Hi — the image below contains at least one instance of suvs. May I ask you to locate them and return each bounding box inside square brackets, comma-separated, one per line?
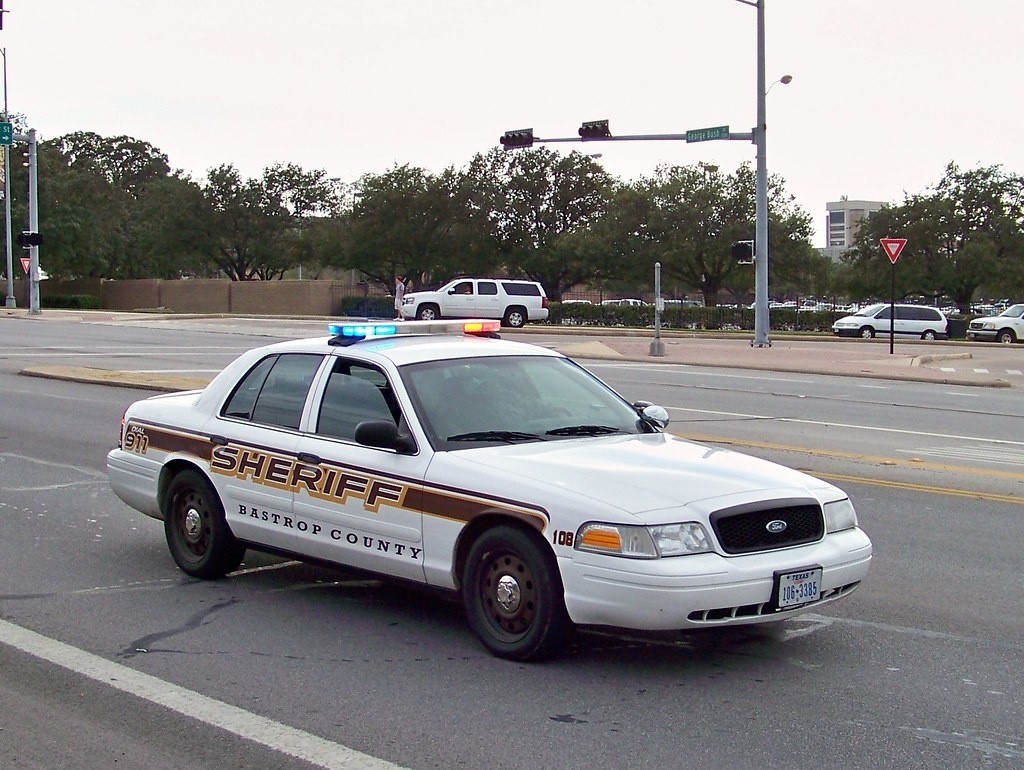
[400, 278, 550, 328]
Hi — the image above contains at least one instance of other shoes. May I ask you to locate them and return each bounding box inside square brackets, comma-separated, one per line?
[393, 317, 400, 321]
[399, 318, 405, 322]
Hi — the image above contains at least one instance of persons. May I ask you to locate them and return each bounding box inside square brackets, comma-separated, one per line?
[392, 277, 406, 323]
[432, 364, 572, 439]
[463, 284, 472, 294]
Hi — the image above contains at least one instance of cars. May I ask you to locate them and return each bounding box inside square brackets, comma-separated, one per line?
[105, 324, 873, 665]
[562, 297, 1012, 319]
[966, 302, 1024, 345]
[831, 303, 949, 342]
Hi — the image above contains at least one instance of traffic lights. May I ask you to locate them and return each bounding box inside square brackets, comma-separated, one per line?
[578, 119, 609, 140]
[500, 128, 535, 150]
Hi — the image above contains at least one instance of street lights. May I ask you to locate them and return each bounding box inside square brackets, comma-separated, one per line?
[753, 75, 793, 348]
